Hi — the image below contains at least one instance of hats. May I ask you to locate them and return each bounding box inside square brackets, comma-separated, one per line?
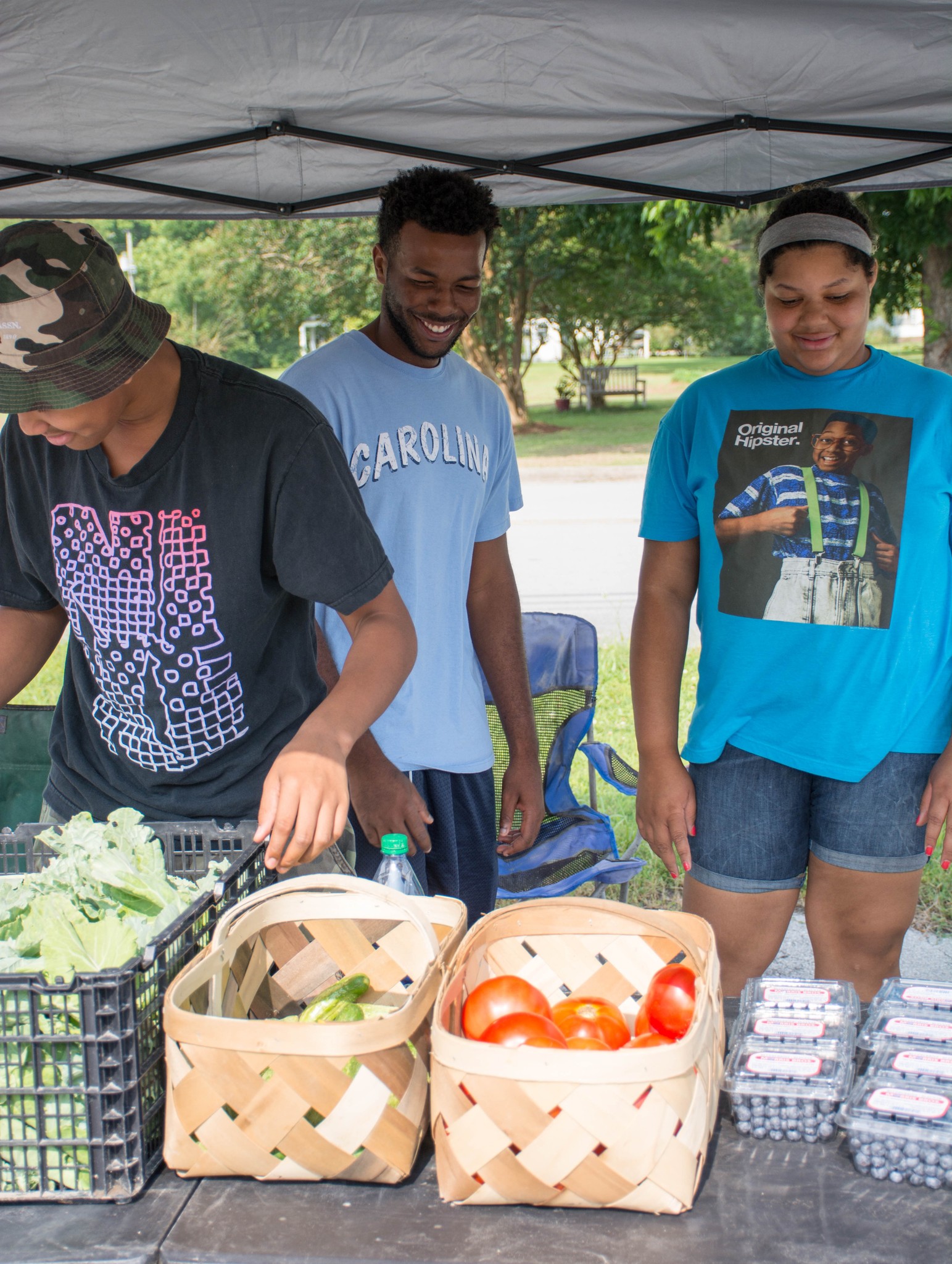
[0, 220, 171, 414]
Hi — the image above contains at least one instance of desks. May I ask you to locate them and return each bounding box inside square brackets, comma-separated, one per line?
[0, 994, 952, 1264]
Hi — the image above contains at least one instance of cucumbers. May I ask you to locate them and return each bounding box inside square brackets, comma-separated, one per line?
[260, 973, 419, 1160]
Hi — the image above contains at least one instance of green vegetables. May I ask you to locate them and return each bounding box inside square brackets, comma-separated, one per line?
[0, 807, 265, 1197]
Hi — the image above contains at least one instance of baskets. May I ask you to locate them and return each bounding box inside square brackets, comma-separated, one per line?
[160, 873, 469, 1184]
[428, 896, 726, 1215]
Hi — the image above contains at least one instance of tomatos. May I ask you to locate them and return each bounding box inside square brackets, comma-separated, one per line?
[456, 965, 697, 1190]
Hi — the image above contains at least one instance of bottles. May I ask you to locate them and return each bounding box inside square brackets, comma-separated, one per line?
[371, 833, 425, 898]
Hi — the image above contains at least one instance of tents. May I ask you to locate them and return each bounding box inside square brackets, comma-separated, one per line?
[0, 0, 952, 218]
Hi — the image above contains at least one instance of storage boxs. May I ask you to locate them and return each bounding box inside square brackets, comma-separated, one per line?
[0, 821, 276, 1214]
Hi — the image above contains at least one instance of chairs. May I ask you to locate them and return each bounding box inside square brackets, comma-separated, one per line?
[474, 612, 645, 904]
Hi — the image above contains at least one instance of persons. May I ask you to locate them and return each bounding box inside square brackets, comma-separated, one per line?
[277, 165, 542, 930]
[629, 182, 952, 1001]
[0, 222, 417, 884]
